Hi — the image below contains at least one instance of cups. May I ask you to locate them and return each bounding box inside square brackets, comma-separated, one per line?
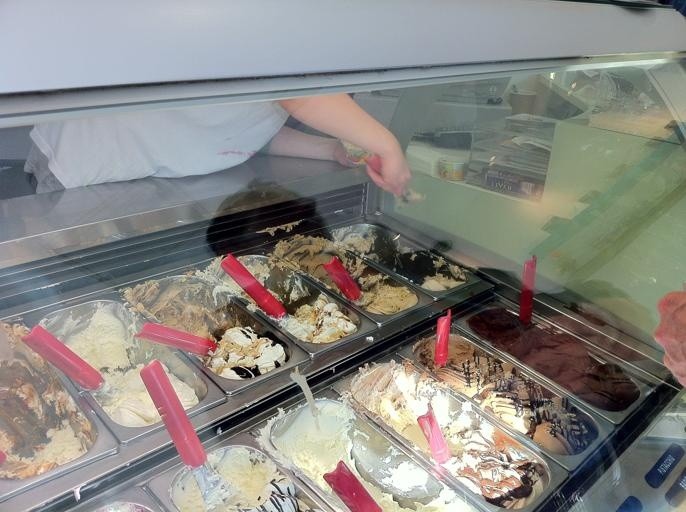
[508, 87, 538, 117]
[438, 151, 467, 184]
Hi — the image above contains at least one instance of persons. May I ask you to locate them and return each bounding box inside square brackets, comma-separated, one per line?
[653, 283, 686, 390]
[22, 87, 414, 210]
